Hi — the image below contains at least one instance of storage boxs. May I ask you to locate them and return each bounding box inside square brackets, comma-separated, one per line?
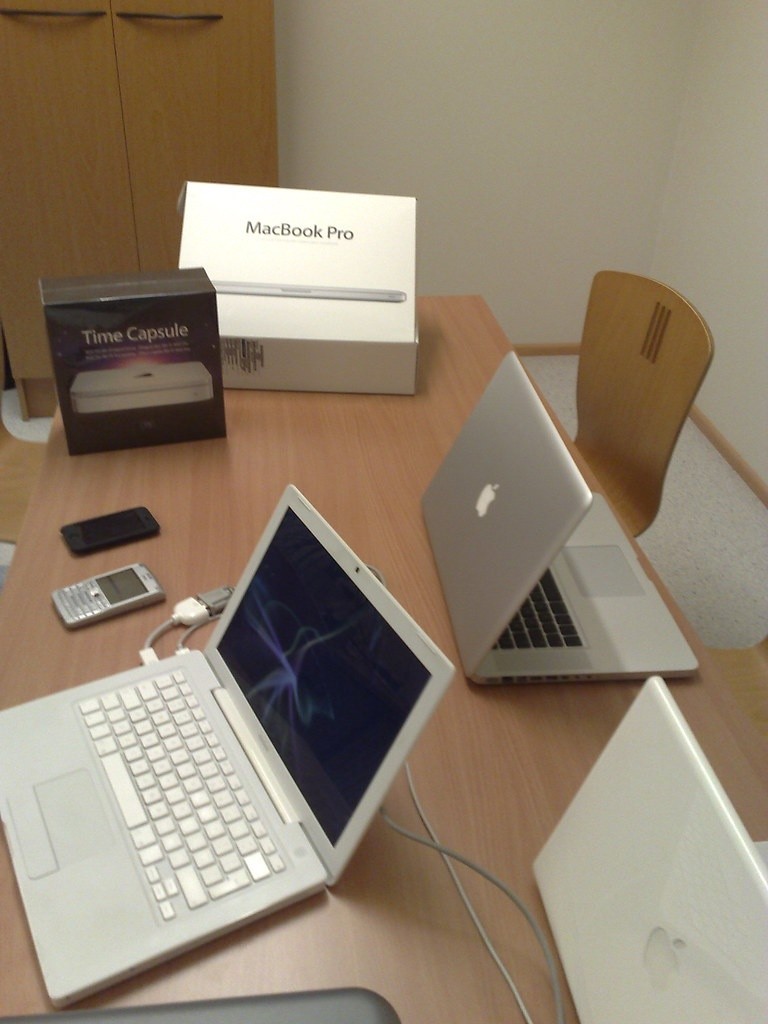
[38, 267, 226, 457]
[177, 181, 420, 396]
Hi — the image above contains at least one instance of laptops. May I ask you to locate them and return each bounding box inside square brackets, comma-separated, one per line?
[421, 351, 699, 686]
[533, 675, 768, 1024]
[0, 484, 456, 1008]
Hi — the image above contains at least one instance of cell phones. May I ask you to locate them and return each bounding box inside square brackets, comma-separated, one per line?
[60, 507, 160, 554]
[51, 563, 165, 629]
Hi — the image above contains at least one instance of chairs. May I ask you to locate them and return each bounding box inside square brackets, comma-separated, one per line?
[702, 633, 768, 762]
[573, 271, 715, 539]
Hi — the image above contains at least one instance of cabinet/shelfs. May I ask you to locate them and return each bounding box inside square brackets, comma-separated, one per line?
[0, 0, 281, 422]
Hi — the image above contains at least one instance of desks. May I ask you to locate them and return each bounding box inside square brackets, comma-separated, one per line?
[0, 294, 768, 1024]
